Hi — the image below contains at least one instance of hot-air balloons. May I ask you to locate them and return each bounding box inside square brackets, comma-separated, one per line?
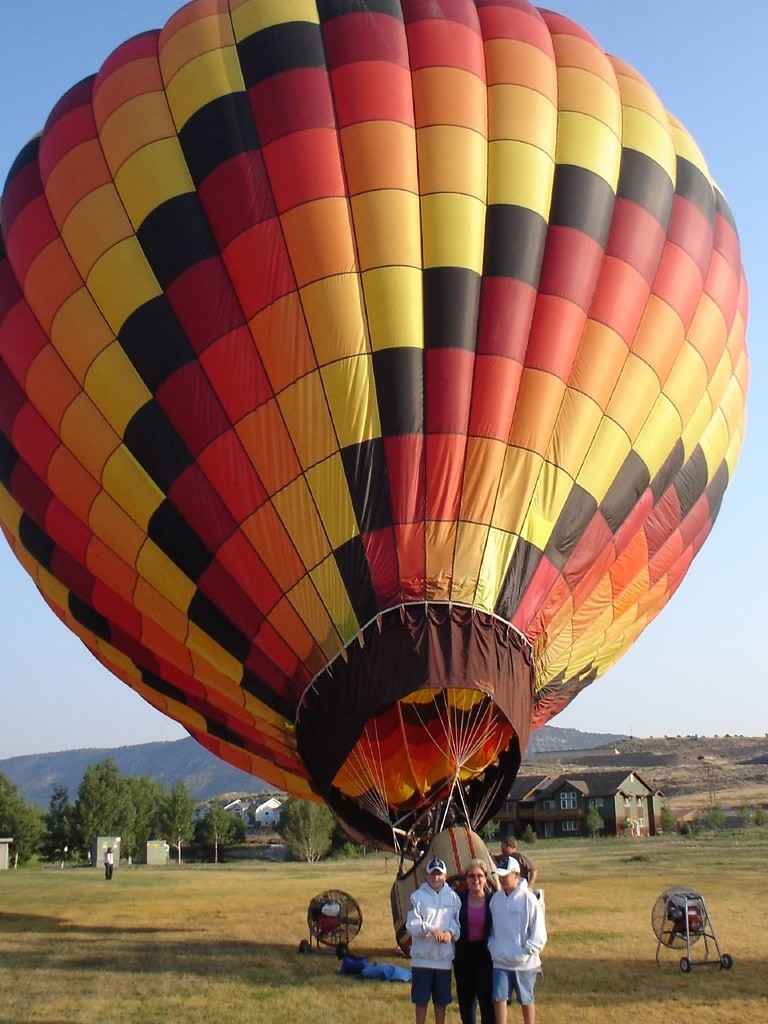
[0, 0, 747, 961]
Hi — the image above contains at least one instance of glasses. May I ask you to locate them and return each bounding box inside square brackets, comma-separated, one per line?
[469, 874, 483, 878]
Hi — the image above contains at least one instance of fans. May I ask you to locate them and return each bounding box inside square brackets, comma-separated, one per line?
[303, 888, 364, 957]
[650, 886, 735, 970]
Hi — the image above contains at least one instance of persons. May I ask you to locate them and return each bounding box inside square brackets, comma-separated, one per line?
[104, 847, 114, 880]
[404, 858, 462, 1024]
[486, 856, 548, 1024]
[452, 859, 494, 1024]
[495, 835, 538, 885]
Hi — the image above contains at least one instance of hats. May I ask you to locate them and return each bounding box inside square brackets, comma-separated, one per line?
[490, 856, 521, 877]
[425, 858, 447, 874]
